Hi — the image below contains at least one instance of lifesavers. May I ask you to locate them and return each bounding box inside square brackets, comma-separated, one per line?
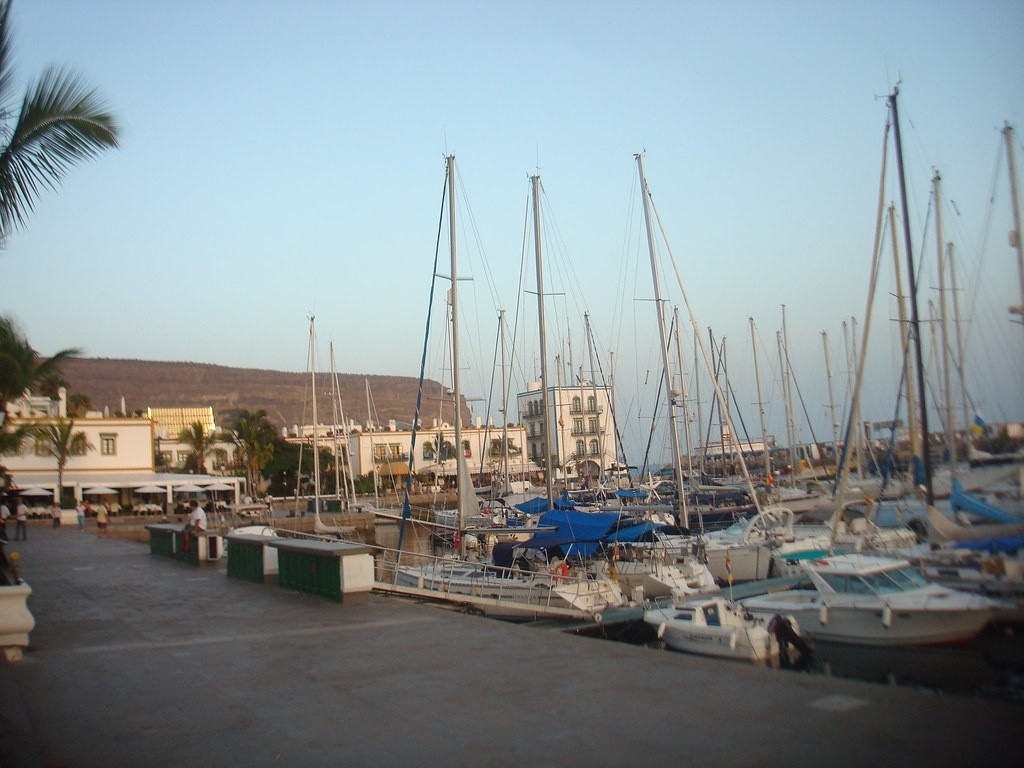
[552, 561, 569, 582]
[809, 559, 829, 566]
[453, 531, 461, 549]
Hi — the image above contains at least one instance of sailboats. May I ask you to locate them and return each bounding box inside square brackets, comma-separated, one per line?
[286, 78, 1022, 650]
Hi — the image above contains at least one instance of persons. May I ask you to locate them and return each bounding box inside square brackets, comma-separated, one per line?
[74, 502, 87, 534]
[50, 503, 60, 536]
[264, 493, 273, 513]
[0, 501, 11, 544]
[97, 502, 112, 542]
[12, 497, 30, 542]
[181, 500, 207, 555]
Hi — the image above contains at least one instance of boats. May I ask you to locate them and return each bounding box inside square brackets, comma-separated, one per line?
[642, 595, 821, 672]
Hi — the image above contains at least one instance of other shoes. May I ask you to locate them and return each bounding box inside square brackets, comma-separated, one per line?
[181, 550, 190, 554]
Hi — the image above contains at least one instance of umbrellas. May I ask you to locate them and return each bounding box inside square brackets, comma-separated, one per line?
[173, 484, 235, 513]
[81, 487, 118, 496]
[134, 485, 167, 505]
[19, 488, 53, 497]
